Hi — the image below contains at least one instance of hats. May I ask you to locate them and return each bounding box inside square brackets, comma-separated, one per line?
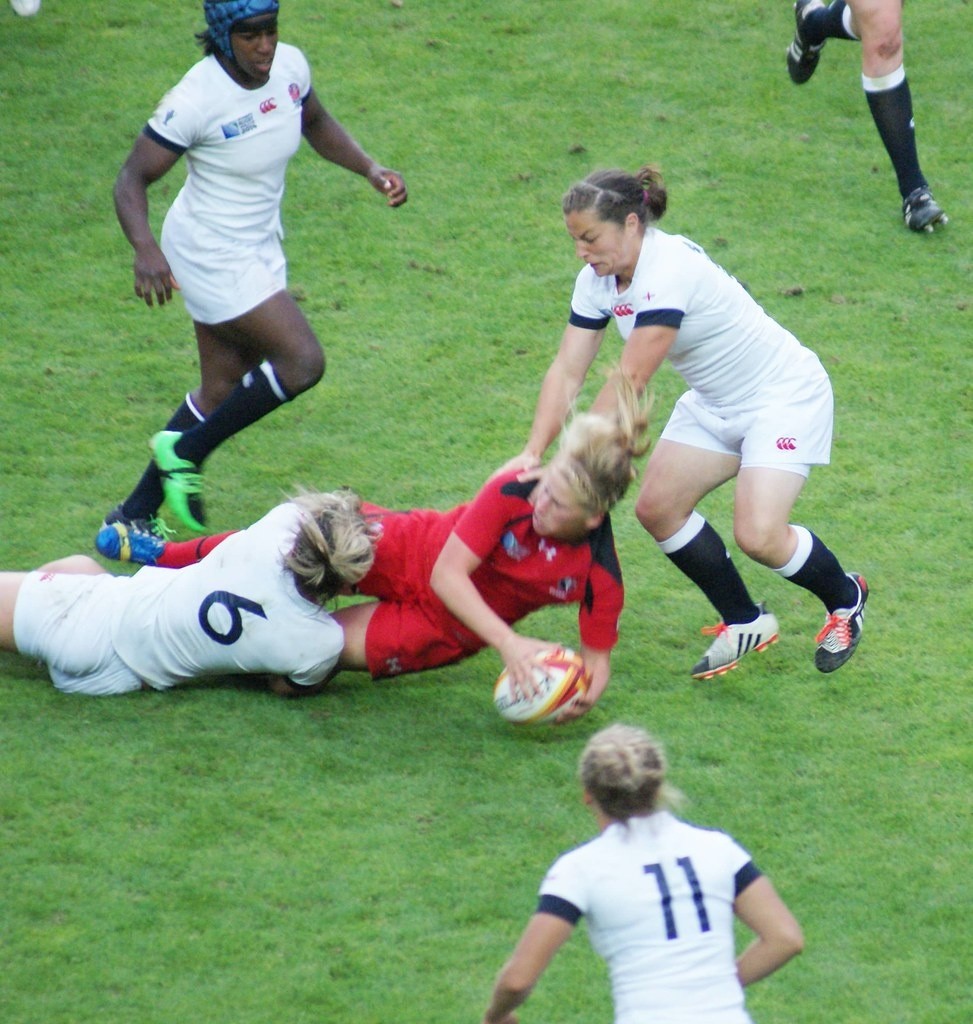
[203, 0, 280, 62]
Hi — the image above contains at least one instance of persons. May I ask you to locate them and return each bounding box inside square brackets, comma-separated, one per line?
[99, 0, 408, 541]
[481, 722, 804, 1024]
[787, 0, 947, 234]
[482, 164, 869, 681]
[0, 489, 378, 700]
[94, 365, 653, 726]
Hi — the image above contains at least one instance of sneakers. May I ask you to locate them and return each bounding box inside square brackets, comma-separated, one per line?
[95, 502, 171, 567]
[148, 430, 207, 533]
[815, 572, 868, 673]
[902, 183, 949, 233]
[786, 0, 827, 84]
[691, 600, 781, 680]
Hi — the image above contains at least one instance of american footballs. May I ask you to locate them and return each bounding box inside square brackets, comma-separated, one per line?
[493, 645, 593, 726]
